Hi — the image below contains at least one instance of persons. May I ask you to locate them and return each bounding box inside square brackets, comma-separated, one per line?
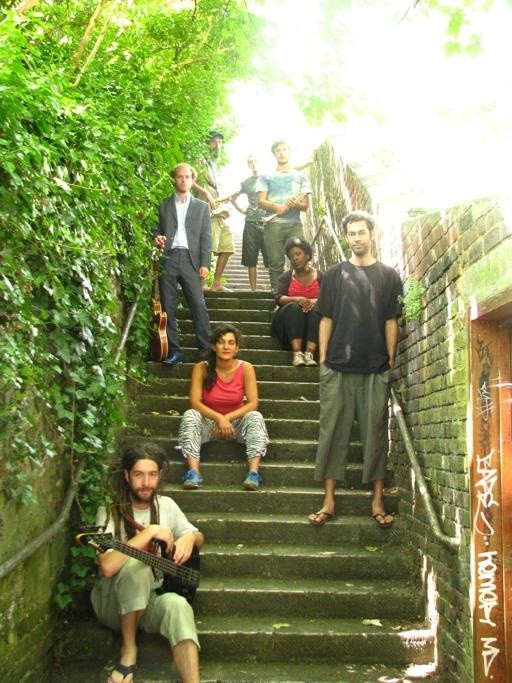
[253, 137, 313, 293]
[178, 323, 268, 489]
[150, 162, 215, 366]
[231, 153, 271, 291]
[308, 210, 404, 527]
[272, 236, 326, 367]
[194, 128, 234, 292]
[90, 443, 205, 683]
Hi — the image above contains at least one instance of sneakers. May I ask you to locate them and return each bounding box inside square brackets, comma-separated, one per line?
[303, 352, 318, 367]
[182, 469, 204, 489]
[241, 472, 263, 490]
[291, 351, 306, 367]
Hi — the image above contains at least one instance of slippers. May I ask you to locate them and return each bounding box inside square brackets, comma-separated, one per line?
[370, 512, 392, 528]
[106, 651, 142, 683]
[309, 511, 337, 525]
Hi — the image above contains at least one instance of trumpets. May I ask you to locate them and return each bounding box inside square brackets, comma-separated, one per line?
[260, 190, 311, 226]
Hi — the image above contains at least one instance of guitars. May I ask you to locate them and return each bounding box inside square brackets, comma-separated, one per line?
[149, 235, 169, 361]
[207, 193, 237, 214]
[75, 532, 201, 603]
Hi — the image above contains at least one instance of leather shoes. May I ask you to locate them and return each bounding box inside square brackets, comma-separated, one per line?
[162, 353, 183, 365]
